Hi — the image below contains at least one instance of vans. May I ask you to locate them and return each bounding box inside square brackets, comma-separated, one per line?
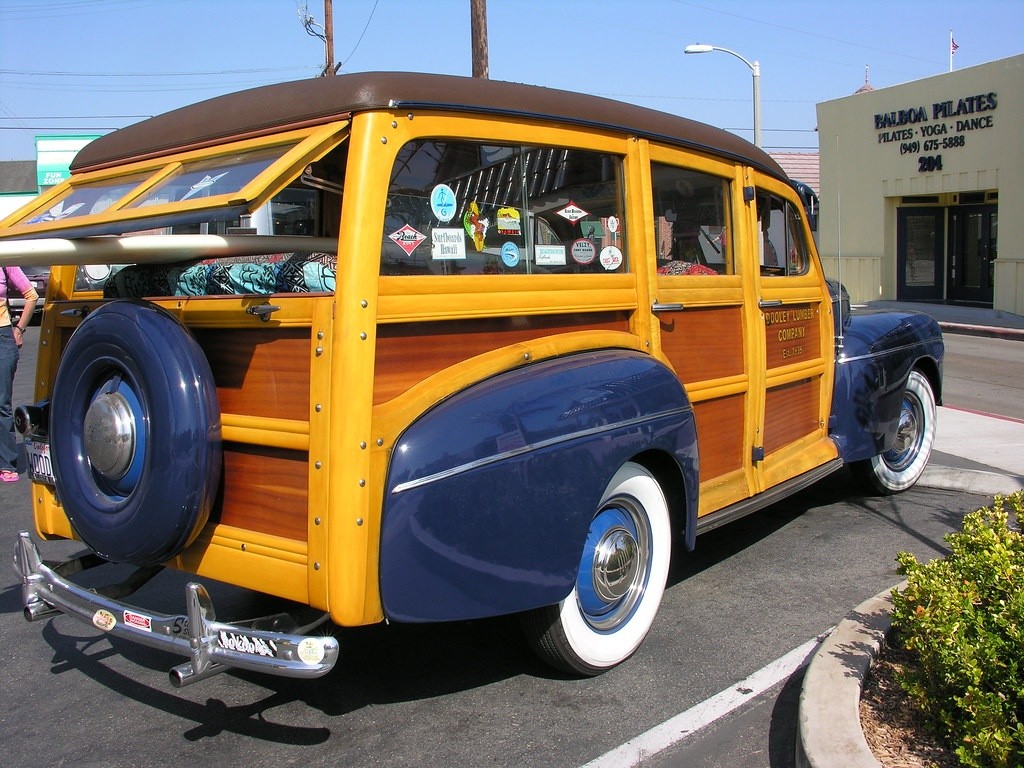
[1, 68, 949, 690]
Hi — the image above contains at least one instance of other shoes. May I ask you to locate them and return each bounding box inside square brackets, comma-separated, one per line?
[0, 470, 20, 482]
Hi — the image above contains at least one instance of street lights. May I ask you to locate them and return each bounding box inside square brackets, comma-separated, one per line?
[684, 43, 761, 149]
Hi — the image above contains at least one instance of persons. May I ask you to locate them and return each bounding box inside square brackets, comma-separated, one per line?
[0, 263, 40, 482]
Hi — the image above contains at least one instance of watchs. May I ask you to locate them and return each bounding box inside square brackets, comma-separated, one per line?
[16, 325, 26, 333]
